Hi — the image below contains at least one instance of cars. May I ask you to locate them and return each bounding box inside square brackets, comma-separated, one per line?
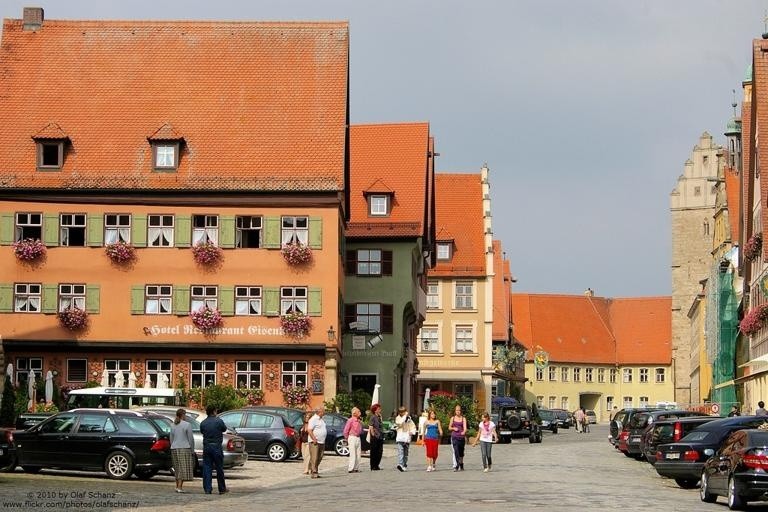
[699, 429, 767, 509]
[608, 407, 767, 489]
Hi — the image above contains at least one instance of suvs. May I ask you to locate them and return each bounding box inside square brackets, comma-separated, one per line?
[538, 408, 575, 433]
[497, 402, 543, 443]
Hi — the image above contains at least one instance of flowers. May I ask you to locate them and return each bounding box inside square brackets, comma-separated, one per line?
[739, 303, 768, 337]
[742, 232, 764, 261]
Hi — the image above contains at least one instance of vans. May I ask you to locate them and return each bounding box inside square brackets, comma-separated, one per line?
[585, 410, 596, 424]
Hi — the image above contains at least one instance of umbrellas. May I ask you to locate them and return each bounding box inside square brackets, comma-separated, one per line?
[28, 370, 37, 410]
[100, 369, 169, 406]
[423, 388, 431, 412]
[45, 371, 53, 402]
[371, 384, 381, 406]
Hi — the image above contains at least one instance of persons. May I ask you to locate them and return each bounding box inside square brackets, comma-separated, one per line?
[757, 419, 768, 429]
[169, 409, 195, 493]
[448, 405, 467, 472]
[728, 406, 740, 417]
[756, 401, 768, 416]
[422, 410, 443, 472]
[343, 407, 363, 473]
[572, 406, 590, 433]
[472, 413, 499, 473]
[369, 404, 384, 470]
[300, 407, 327, 478]
[199, 404, 229, 494]
[394, 406, 417, 472]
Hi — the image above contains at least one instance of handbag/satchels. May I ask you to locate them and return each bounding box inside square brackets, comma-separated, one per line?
[365, 430, 371, 443]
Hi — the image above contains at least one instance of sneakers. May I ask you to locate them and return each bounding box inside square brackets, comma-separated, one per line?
[303, 463, 498, 479]
[173, 486, 228, 495]
